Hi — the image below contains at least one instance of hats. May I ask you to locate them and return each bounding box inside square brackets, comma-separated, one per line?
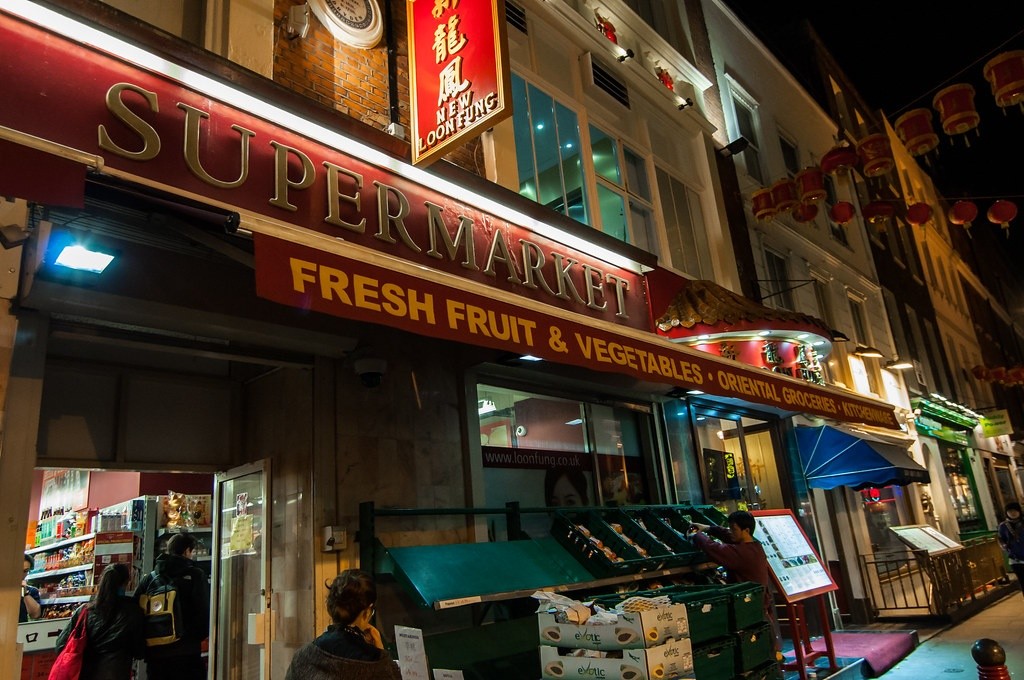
[1005, 502, 1021, 514]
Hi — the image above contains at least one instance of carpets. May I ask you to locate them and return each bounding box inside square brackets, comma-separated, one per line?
[784, 633, 916, 678]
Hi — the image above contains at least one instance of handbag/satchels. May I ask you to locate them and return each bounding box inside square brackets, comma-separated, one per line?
[48, 606, 87, 680]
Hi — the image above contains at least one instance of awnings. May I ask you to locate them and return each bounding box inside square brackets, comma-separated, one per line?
[793, 425, 932, 492]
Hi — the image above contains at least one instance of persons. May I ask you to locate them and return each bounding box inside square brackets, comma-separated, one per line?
[55, 562, 146, 680]
[997, 502, 1024, 597]
[133, 535, 210, 680]
[688, 510, 784, 680]
[284, 569, 402, 680]
[18, 553, 41, 623]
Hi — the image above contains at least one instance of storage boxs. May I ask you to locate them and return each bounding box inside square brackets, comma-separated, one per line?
[651, 506, 714, 565]
[532, 581, 783, 680]
[550, 509, 643, 579]
[590, 507, 674, 573]
[624, 507, 700, 569]
[675, 506, 722, 540]
[694, 504, 762, 545]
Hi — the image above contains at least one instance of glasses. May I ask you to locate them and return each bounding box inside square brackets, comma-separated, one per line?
[23, 570, 30, 575]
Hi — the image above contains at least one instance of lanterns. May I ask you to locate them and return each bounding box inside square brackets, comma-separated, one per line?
[972, 365, 1024, 387]
[752, 50, 1024, 219]
[793, 198, 1017, 239]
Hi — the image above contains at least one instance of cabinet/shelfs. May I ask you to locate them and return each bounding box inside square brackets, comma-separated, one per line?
[22, 494, 214, 680]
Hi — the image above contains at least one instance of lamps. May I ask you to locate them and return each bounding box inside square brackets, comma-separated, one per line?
[677, 98, 694, 110]
[717, 136, 750, 159]
[618, 49, 636, 63]
[282, 1, 310, 39]
[387, 123, 404, 139]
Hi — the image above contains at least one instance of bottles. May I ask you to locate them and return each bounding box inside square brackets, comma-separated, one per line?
[34, 504, 84, 548]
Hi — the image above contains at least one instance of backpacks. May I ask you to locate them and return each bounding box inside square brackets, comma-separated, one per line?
[140, 570, 185, 648]
